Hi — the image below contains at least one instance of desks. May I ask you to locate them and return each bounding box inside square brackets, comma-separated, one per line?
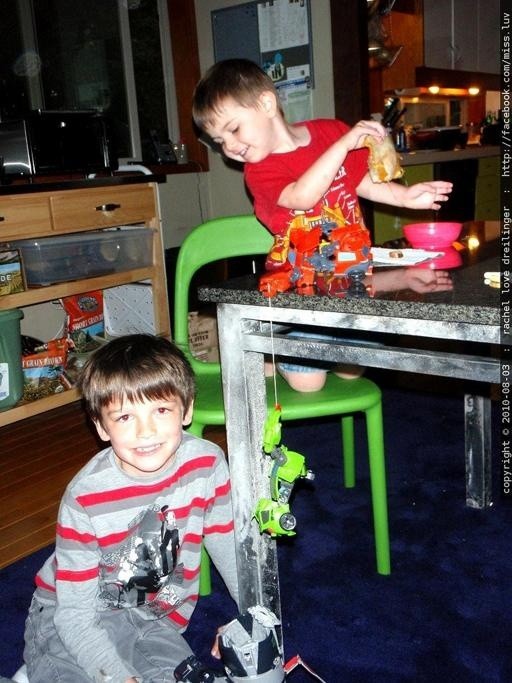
[198, 218, 512, 681]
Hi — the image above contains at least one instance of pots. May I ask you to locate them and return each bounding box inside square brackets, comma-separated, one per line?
[410, 124, 462, 153]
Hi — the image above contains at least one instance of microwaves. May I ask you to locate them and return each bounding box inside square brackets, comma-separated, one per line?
[0, 107, 121, 183]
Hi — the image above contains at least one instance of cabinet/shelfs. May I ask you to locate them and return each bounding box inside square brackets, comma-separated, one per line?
[478, 0, 500, 74]
[474, 155, 500, 221]
[373, 164, 436, 243]
[422, 0, 478, 71]
[0, 174, 172, 427]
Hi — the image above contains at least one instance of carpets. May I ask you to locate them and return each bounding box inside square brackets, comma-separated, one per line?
[2, 365, 511, 683]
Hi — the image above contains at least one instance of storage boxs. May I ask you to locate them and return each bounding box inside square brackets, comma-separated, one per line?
[0, 308, 25, 410]
[8, 225, 157, 289]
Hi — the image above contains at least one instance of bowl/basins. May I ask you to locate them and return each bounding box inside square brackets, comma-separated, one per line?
[406, 245, 463, 270]
[402, 220, 463, 248]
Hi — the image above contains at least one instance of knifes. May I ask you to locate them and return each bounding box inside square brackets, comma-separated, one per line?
[384, 96, 408, 128]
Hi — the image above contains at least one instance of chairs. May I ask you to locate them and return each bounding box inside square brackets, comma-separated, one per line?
[176, 216, 395, 595]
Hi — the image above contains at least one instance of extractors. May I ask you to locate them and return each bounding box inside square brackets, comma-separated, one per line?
[395, 87, 479, 97]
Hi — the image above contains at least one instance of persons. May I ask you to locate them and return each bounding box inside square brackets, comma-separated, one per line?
[0, 327, 239, 683]
[186, 50, 454, 394]
[365, 269, 455, 295]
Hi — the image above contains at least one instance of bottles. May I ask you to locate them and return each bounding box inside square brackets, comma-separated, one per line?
[485, 110, 498, 125]
